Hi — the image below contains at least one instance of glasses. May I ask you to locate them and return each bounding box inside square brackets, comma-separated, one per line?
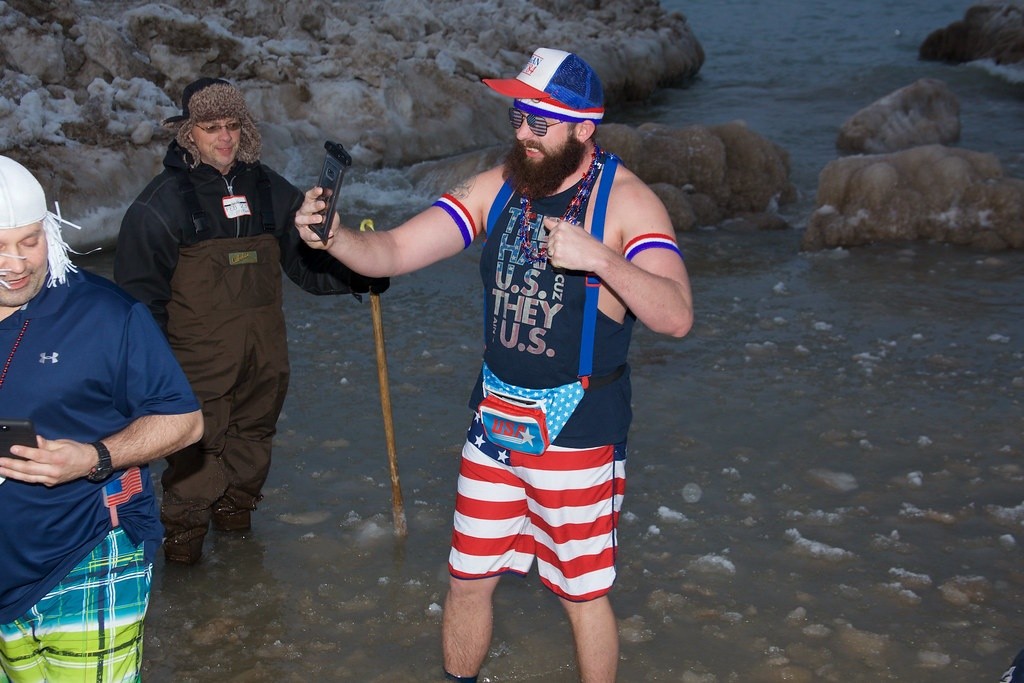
[195, 123, 241, 134]
[508, 107, 563, 136]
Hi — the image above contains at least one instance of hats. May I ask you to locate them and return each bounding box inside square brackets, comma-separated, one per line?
[482, 47, 605, 123]
[175, 78, 262, 169]
[0, 155, 102, 290]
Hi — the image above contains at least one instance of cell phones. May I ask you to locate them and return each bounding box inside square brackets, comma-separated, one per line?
[0, 420, 38, 461]
[308, 141, 352, 246]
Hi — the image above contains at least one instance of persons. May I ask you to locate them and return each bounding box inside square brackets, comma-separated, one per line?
[0, 156, 203, 682]
[292, 48, 697, 683]
[111, 73, 395, 569]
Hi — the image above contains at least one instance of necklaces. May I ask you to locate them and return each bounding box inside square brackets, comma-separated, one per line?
[516, 140, 606, 264]
[0, 305, 33, 388]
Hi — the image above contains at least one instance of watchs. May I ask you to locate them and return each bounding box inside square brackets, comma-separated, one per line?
[89, 441, 113, 481]
